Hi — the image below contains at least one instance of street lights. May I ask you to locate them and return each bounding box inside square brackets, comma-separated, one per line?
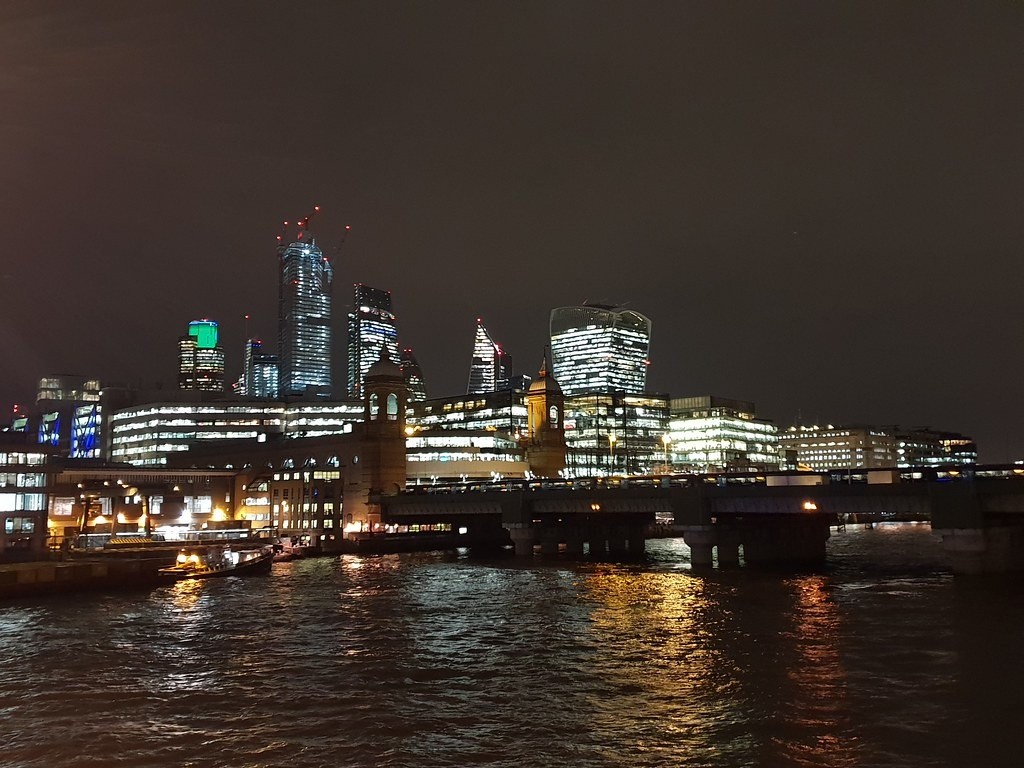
[609, 436, 616, 478]
[662, 434, 672, 473]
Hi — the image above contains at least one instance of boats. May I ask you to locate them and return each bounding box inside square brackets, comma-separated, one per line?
[158, 541, 276, 583]
